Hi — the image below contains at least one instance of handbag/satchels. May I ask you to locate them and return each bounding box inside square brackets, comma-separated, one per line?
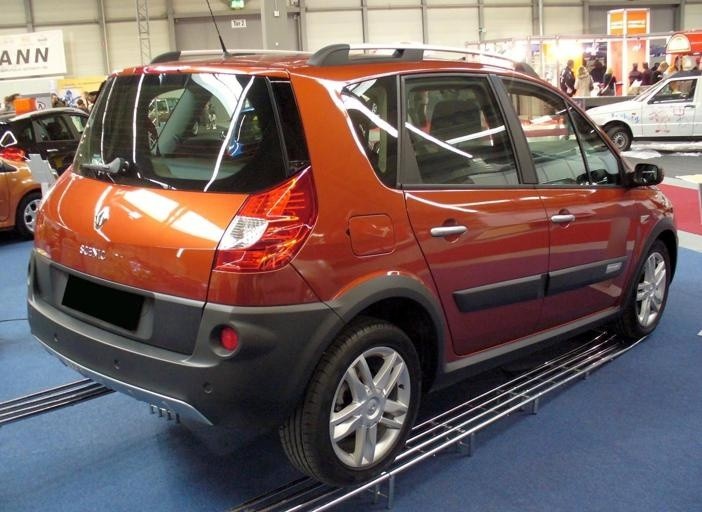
[566, 86, 577, 96]
[597, 88, 609, 96]
[589, 75, 595, 91]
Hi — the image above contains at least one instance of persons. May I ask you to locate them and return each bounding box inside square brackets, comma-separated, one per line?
[78, 99, 89, 129]
[628, 60, 701, 94]
[67, 90, 82, 108]
[51, 93, 69, 108]
[561, 57, 616, 124]
